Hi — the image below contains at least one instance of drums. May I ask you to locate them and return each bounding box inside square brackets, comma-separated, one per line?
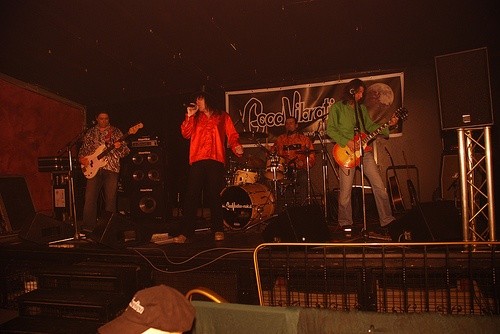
[264, 154, 287, 180]
[226, 159, 239, 182]
[234, 169, 259, 186]
[220, 184, 275, 230]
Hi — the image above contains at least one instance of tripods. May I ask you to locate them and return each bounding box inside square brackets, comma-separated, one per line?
[344, 94, 393, 244]
[48, 125, 96, 245]
[301, 155, 314, 206]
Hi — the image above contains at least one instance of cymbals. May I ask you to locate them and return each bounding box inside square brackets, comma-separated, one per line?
[237, 130, 269, 140]
[289, 113, 329, 135]
[296, 150, 320, 154]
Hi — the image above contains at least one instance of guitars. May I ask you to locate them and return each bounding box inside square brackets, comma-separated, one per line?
[332, 107, 408, 169]
[384, 147, 405, 210]
[80, 123, 144, 179]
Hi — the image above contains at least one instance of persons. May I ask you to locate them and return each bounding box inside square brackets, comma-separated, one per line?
[97, 284, 197, 334]
[327, 78, 397, 232]
[78, 111, 130, 233]
[271, 116, 317, 171]
[173, 92, 244, 244]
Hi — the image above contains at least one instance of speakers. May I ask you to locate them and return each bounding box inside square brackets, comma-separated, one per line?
[438, 154, 487, 203]
[262, 204, 331, 251]
[90, 155, 171, 247]
[19, 213, 76, 242]
[434, 47, 500, 132]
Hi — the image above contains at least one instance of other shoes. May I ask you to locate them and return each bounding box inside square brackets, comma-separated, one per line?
[387, 221, 406, 232]
[83, 226, 96, 232]
[341, 224, 354, 239]
[172, 234, 187, 242]
[103, 217, 113, 228]
[215, 231, 224, 240]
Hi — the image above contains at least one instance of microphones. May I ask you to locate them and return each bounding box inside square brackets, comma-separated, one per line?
[288, 129, 292, 135]
[183, 103, 195, 107]
[86, 120, 96, 127]
[350, 89, 358, 94]
[311, 122, 321, 143]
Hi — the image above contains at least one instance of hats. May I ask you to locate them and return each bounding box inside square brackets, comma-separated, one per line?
[98, 285, 195, 334]
